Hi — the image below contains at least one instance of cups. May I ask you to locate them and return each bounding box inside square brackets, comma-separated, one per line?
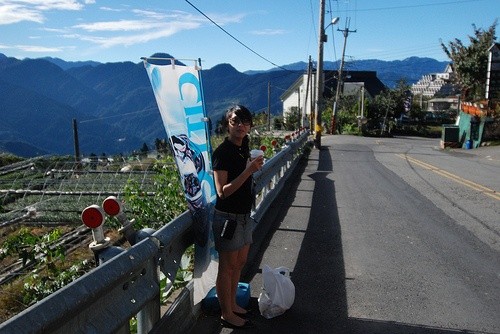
[250, 150, 263, 161]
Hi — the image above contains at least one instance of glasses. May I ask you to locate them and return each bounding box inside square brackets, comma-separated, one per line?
[229, 119, 251, 126]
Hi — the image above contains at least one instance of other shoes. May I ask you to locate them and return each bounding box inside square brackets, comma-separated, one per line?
[220, 315, 249, 332]
[232, 304, 253, 319]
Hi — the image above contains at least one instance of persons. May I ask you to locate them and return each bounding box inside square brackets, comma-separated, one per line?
[211, 104, 265, 331]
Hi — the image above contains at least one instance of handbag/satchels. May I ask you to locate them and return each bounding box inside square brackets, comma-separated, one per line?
[256, 262, 296, 320]
